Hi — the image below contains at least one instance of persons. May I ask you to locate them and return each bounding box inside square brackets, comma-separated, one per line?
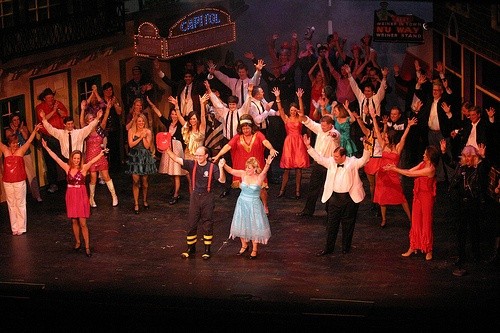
[158, 63, 209, 197]
[79, 82, 122, 207]
[38, 108, 103, 168]
[221, 154, 274, 257]
[394, 58, 496, 203]
[438, 137, 500, 275]
[214, 114, 278, 216]
[37, 87, 68, 192]
[324, 30, 417, 229]
[169, 147, 226, 259]
[123, 66, 156, 214]
[5, 112, 43, 202]
[203, 53, 282, 196]
[386, 146, 440, 259]
[242, 28, 340, 217]
[145, 94, 189, 204]
[301, 135, 372, 256]
[41, 138, 109, 258]
[0, 123, 42, 236]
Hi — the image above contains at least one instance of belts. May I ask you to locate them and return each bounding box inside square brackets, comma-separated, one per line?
[433, 130, 439, 132]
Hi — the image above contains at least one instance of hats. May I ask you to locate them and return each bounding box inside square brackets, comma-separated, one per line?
[132, 66, 142, 71]
[39, 88, 57, 100]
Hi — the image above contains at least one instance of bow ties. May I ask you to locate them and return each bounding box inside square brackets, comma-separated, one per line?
[337, 163, 344, 167]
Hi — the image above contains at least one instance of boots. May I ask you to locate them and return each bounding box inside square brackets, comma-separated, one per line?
[89, 183, 97, 208]
[104, 180, 118, 207]
[181, 235, 213, 260]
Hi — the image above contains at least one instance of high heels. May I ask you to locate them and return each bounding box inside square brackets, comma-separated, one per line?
[402, 247, 432, 260]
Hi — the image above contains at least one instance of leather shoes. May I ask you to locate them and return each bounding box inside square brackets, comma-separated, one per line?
[342, 249, 350, 254]
[316, 250, 329, 256]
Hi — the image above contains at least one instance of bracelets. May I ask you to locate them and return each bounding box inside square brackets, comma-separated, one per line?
[174, 107, 179, 110]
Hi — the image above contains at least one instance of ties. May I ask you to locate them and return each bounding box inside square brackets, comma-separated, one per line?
[69, 132, 72, 158]
[230, 111, 233, 140]
[241, 81, 244, 105]
[365, 98, 370, 125]
[261, 103, 268, 128]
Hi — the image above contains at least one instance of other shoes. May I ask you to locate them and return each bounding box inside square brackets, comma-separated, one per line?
[169, 188, 177, 205]
[222, 188, 313, 261]
[48, 185, 58, 192]
[453, 267, 466, 277]
[85, 249, 92, 256]
[380, 220, 387, 230]
[73, 244, 81, 253]
[135, 208, 138, 214]
[143, 201, 149, 209]
[37, 196, 43, 202]
